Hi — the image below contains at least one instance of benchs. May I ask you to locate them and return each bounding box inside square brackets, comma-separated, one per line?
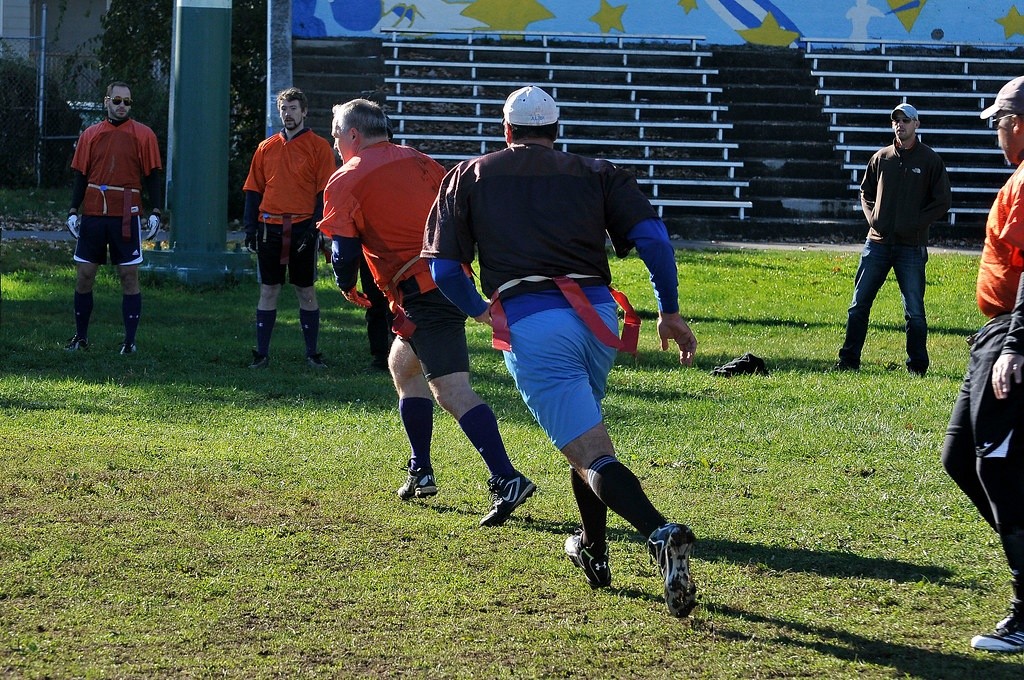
[799, 36, 1024, 227]
[382, 27, 754, 219]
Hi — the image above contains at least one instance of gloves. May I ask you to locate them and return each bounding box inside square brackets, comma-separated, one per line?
[295, 230, 314, 256]
[245, 233, 257, 254]
[340, 285, 372, 309]
[145, 213, 160, 241]
[65, 212, 80, 240]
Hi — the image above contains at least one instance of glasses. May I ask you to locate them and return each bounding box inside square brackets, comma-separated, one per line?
[105, 96, 134, 106]
[987, 114, 1014, 129]
[893, 116, 911, 123]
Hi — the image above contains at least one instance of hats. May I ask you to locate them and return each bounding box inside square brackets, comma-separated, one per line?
[979, 76, 1024, 119]
[891, 103, 918, 121]
[502, 84, 560, 125]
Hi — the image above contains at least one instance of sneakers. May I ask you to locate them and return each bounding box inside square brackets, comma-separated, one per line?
[565, 534, 613, 589]
[647, 521, 696, 618]
[119, 343, 135, 356]
[307, 353, 328, 370]
[397, 464, 438, 500]
[249, 348, 269, 367]
[823, 362, 859, 373]
[62, 333, 90, 352]
[479, 469, 537, 527]
[970, 606, 1024, 652]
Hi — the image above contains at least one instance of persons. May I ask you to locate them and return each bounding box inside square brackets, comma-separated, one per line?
[831, 103, 951, 377]
[315, 98, 538, 527]
[421, 87, 698, 620]
[64, 81, 162, 353]
[941, 74, 1024, 650]
[242, 88, 336, 368]
